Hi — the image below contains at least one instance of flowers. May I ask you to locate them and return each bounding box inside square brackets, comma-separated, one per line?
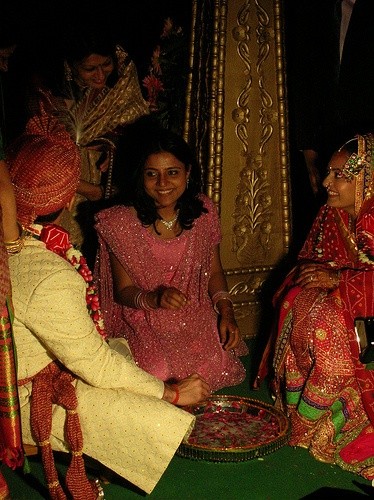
[140, 17, 191, 132]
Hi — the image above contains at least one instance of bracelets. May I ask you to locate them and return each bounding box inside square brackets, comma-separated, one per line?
[329, 268, 340, 287]
[5, 237, 25, 256]
[211, 290, 235, 314]
[170, 383, 180, 405]
[133, 288, 157, 314]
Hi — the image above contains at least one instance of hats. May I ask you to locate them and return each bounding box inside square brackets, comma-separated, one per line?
[9, 112, 82, 228]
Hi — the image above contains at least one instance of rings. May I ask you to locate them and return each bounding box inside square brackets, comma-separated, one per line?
[309, 274, 314, 280]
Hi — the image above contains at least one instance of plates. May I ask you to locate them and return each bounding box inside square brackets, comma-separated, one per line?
[175, 395, 290, 463]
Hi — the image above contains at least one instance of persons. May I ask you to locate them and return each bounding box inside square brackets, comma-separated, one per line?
[91, 138, 249, 393]
[252, 132, 374, 488]
[5, 112, 211, 500]
[298, 0, 374, 209]
[28, 30, 152, 201]
[0, 42, 25, 500]
